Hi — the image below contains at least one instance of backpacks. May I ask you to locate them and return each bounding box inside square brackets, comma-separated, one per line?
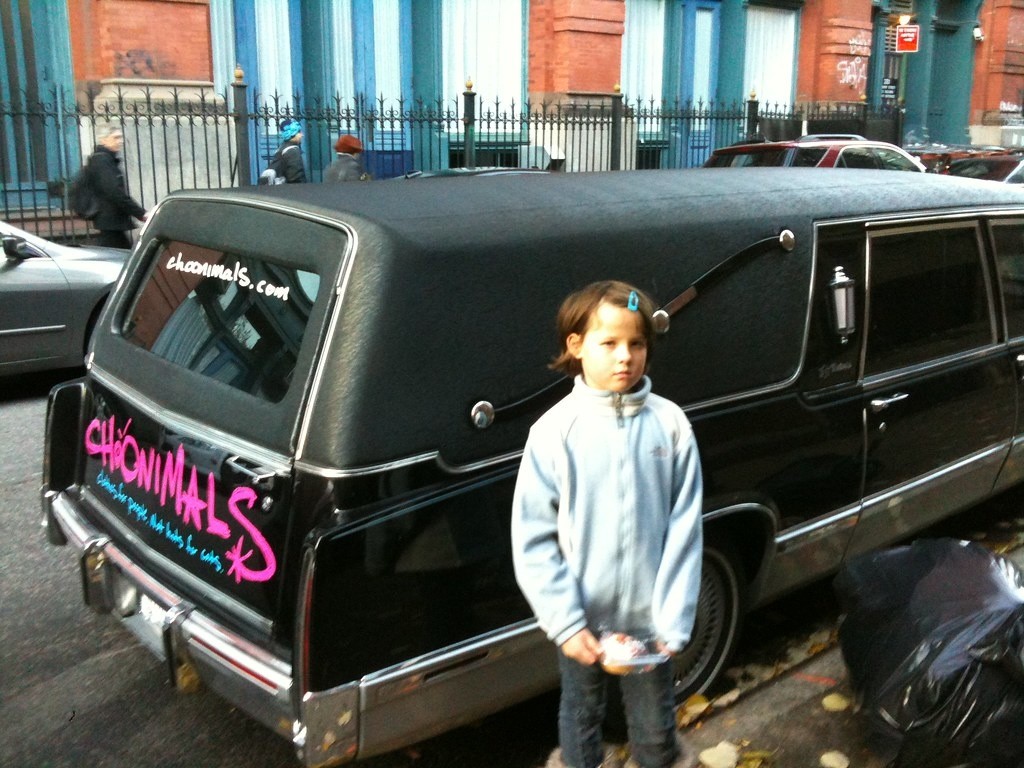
[69, 151, 111, 221]
[259, 145, 300, 185]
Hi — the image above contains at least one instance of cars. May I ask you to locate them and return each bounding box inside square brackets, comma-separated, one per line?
[704, 134, 1024, 199]
[0, 222, 131, 382]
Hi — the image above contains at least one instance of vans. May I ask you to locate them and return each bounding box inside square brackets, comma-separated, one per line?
[39, 157, 1024, 768]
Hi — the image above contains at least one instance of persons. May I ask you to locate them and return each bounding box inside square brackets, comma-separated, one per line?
[322, 134, 365, 184]
[272, 118, 309, 183]
[511, 281, 704, 768]
[87, 121, 148, 250]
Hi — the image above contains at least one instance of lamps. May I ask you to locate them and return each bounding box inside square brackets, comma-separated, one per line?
[890, 15, 911, 27]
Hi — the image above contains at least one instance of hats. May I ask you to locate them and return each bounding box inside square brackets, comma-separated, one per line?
[280, 120, 304, 142]
[334, 135, 364, 155]
